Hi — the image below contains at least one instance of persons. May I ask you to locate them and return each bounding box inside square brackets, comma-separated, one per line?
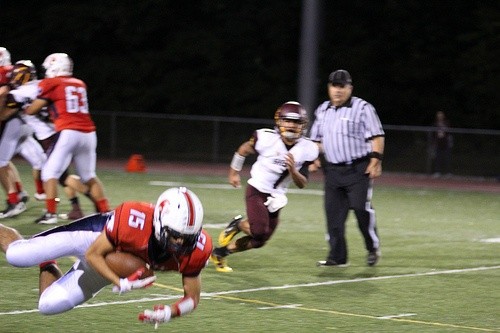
[309, 69, 385, 266]
[0, 187, 215, 326]
[209, 101, 319, 273]
[1, 48, 109, 225]
[429, 110, 454, 177]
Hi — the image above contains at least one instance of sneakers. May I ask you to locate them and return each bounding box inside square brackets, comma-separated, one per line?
[209, 249, 233, 272]
[218, 213, 247, 246]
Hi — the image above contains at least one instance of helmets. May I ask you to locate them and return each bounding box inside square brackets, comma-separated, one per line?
[42, 53, 73, 78]
[0, 47, 36, 86]
[153, 187, 204, 241]
[274, 100, 309, 123]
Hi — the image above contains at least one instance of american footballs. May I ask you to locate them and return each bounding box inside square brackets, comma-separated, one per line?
[104, 251, 153, 283]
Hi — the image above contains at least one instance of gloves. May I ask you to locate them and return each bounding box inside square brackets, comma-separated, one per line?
[139, 305, 172, 329]
[112, 268, 157, 296]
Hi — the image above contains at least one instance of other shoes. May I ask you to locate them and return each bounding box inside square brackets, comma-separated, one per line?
[368, 249, 380, 265]
[17, 190, 29, 203]
[317, 260, 348, 267]
[34, 212, 58, 224]
[58, 209, 83, 219]
[0, 202, 28, 218]
[33, 192, 61, 204]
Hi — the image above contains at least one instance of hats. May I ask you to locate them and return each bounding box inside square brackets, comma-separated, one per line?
[328, 70, 352, 84]
